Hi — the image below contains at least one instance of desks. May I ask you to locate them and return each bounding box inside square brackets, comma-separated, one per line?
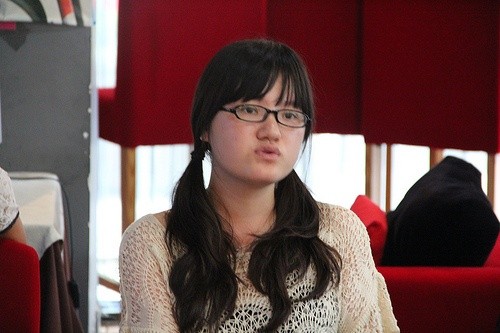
[9, 171, 84, 333]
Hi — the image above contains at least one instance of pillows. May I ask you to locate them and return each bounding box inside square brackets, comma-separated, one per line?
[383, 156, 500, 268]
[349, 195, 389, 265]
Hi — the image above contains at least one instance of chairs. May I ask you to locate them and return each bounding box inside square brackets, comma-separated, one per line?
[99, 0, 500, 293]
[0, 238, 40, 333]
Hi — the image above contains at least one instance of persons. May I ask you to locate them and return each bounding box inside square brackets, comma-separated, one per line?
[118, 38, 400, 333]
[0, 166, 28, 244]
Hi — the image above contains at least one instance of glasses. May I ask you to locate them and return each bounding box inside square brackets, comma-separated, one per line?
[220, 104, 309, 129]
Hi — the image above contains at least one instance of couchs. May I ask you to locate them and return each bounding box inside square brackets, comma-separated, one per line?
[376, 232, 500, 333]
